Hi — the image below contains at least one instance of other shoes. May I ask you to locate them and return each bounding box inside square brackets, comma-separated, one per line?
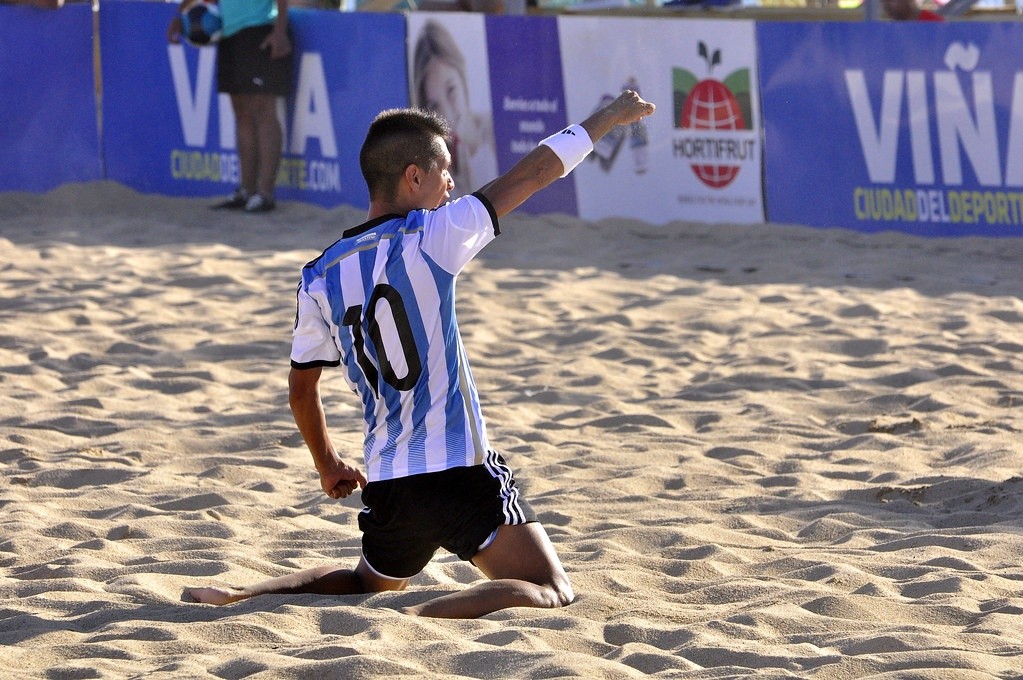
[210, 190, 245, 210]
[244, 192, 275, 212]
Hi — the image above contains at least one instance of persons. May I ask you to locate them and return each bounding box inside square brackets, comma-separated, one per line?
[412, 18, 497, 194]
[169, 0, 294, 212]
[0, 0, 97, 12]
[189, 89, 654, 620]
[289, 0, 1023, 22]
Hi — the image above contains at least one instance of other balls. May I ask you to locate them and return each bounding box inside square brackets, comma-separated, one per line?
[182, 2, 225, 46]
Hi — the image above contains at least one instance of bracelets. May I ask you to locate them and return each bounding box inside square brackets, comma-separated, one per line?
[538, 123, 594, 179]
